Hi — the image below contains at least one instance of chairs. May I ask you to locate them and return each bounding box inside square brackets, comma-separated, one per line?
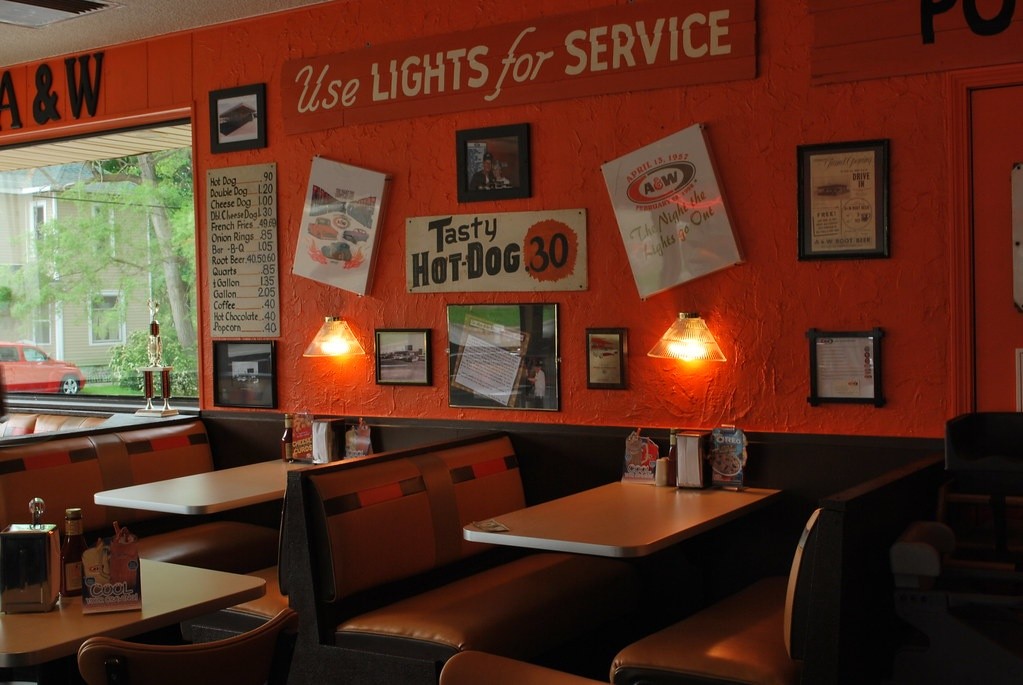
[74, 607, 300, 685]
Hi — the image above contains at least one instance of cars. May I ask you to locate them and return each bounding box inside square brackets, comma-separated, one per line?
[0, 342, 86, 395]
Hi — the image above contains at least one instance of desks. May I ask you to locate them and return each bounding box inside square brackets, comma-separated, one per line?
[0, 557, 266, 667]
[463, 481, 786, 558]
[93, 460, 317, 515]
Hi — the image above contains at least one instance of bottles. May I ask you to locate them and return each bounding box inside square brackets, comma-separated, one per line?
[281, 414, 292, 461]
[655, 428, 679, 487]
[59, 507, 89, 597]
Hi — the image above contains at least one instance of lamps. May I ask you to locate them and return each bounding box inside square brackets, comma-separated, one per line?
[647, 313, 728, 373]
[304, 317, 366, 357]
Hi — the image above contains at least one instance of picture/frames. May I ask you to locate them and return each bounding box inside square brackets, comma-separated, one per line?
[212, 340, 279, 410]
[445, 304, 562, 413]
[374, 326, 433, 385]
[209, 82, 267, 154]
[455, 123, 533, 202]
[805, 327, 887, 410]
[795, 139, 892, 261]
[585, 328, 630, 391]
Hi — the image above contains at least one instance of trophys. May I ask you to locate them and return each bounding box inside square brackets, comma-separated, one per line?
[134, 299, 179, 417]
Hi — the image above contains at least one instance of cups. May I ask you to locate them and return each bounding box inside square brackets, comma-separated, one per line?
[82, 549, 111, 591]
[112, 535, 139, 590]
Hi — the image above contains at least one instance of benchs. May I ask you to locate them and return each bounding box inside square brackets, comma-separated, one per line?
[608, 452, 937, 685]
[179, 429, 629, 685]
[0, 408, 278, 574]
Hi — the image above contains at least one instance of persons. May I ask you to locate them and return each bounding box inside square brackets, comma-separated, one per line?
[470, 152, 511, 189]
[528, 364, 545, 408]
